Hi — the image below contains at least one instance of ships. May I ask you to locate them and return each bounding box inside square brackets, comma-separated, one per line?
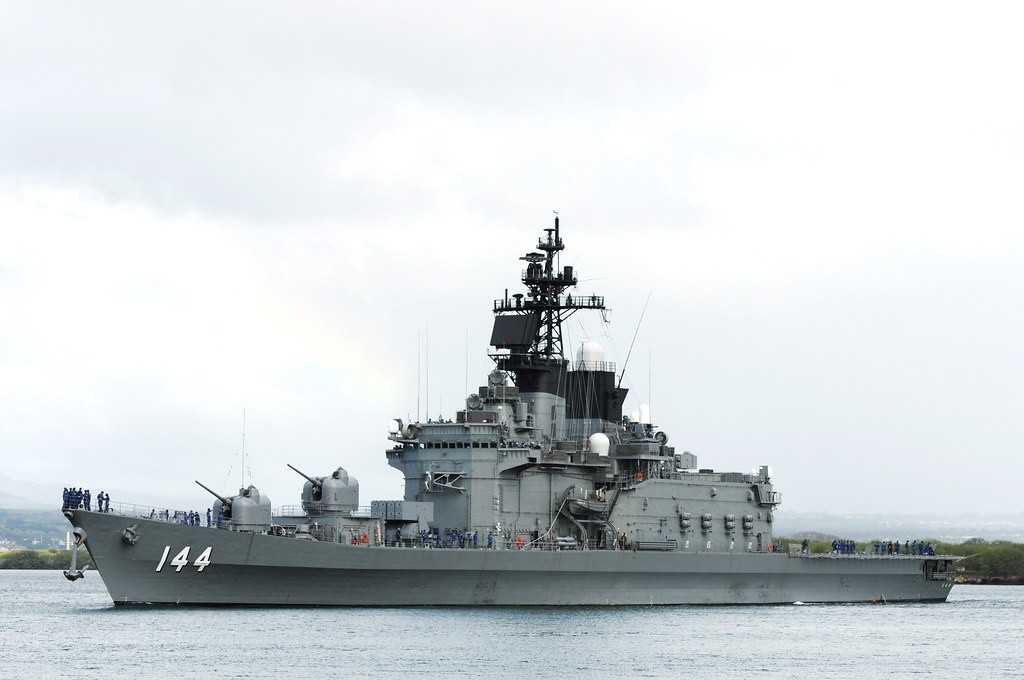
[57, 209, 966, 607]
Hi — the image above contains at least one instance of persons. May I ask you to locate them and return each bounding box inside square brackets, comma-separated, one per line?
[60, 418, 936, 557]
